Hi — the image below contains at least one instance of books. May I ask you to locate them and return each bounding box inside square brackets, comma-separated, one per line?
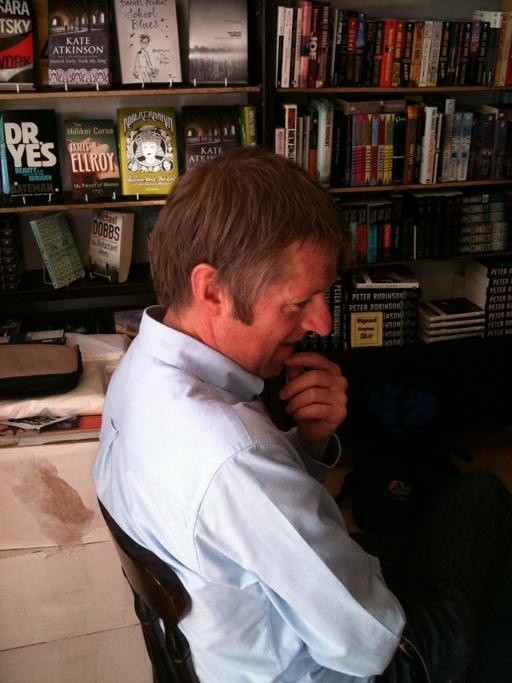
[2, 0, 512, 448]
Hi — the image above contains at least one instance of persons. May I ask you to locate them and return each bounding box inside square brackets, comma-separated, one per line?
[94, 145, 512, 683]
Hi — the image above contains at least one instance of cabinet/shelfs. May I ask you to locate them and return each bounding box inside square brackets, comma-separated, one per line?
[0, 0, 511, 431]
